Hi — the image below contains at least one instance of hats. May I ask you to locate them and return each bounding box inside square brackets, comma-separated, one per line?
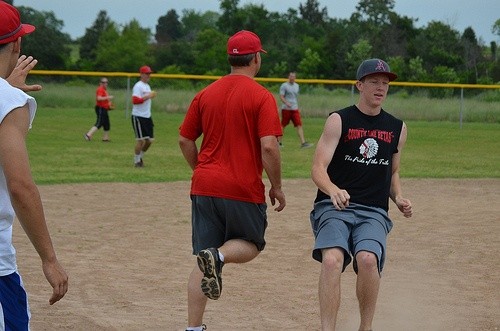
[140, 66, 153, 74]
[357, 58, 398, 82]
[0, 0, 35, 44]
[228, 30, 267, 55]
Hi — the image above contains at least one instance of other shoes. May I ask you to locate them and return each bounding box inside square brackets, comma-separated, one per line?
[301, 142, 312, 149]
[279, 143, 283, 147]
[103, 139, 111, 142]
[84, 134, 90, 141]
[134, 158, 144, 167]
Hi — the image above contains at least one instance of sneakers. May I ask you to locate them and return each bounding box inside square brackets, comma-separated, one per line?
[197, 247, 224, 300]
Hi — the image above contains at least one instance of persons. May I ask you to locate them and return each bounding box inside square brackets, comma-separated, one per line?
[277, 72, 315, 148]
[131, 66, 158, 167]
[310, 59, 412, 331]
[0, 0, 68, 331]
[177, 30, 286, 331]
[83, 78, 114, 142]
